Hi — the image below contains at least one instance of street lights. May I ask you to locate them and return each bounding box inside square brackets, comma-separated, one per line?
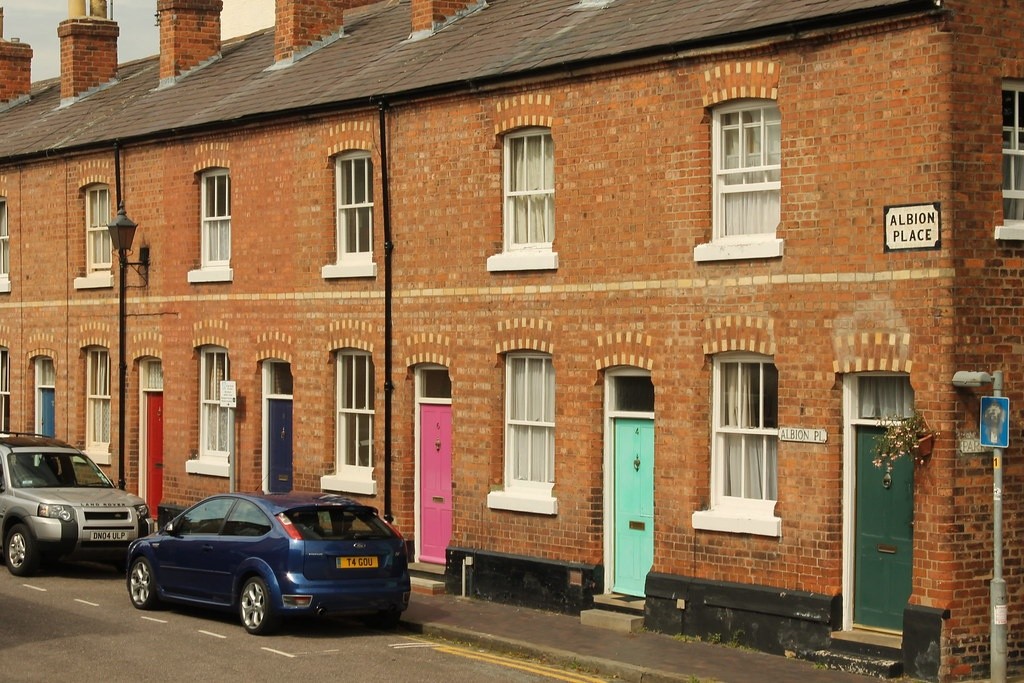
[106, 199, 139, 492]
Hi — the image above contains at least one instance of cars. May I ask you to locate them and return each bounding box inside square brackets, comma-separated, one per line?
[126, 491, 412, 635]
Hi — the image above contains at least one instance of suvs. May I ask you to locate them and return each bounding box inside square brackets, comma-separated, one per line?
[0, 431, 155, 577]
